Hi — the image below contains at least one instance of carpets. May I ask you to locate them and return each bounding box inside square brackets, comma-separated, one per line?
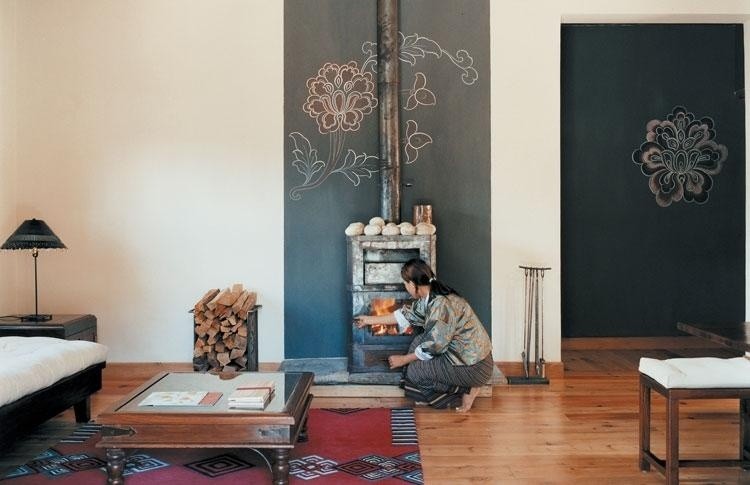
[0, 405, 427, 485]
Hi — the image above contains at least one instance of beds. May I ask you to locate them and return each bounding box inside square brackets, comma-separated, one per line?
[0, 335, 110, 454]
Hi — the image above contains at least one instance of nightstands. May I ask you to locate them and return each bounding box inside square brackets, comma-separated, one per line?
[0, 313, 98, 343]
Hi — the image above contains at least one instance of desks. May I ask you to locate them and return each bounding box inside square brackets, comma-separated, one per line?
[674, 319, 750, 352]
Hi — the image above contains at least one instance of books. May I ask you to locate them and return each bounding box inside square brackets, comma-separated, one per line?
[142, 379, 278, 411]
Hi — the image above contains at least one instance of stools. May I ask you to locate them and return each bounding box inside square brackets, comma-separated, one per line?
[637, 352, 750, 485]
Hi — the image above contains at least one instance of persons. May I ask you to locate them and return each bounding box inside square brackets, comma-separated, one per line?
[352, 256, 495, 415]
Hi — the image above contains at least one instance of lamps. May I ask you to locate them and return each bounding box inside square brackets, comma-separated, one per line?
[0, 217, 69, 322]
